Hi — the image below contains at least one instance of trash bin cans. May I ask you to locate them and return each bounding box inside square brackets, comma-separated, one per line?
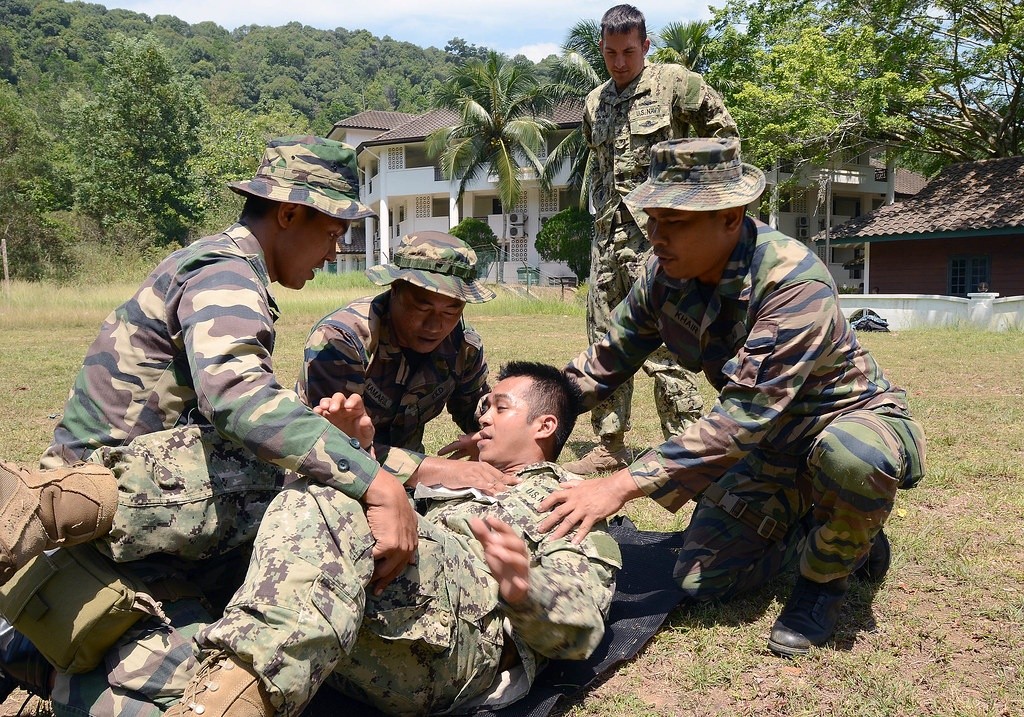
[516, 266, 541, 287]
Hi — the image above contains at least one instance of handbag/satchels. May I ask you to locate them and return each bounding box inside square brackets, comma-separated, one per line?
[1, 545, 155, 676]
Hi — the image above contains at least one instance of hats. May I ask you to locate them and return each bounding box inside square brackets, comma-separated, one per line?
[226, 134, 381, 222]
[364, 230, 497, 303]
[622, 137, 766, 211]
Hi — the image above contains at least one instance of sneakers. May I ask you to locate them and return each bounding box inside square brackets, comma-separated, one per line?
[562, 442, 629, 475]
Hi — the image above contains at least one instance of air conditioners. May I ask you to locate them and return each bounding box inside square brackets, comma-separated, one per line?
[540, 215, 551, 225]
[508, 226, 524, 238]
[797, 216, 810, 226]
[373, 229, 380, 251]
[797, 228, 810, 238]
[509, 213, 524, 224]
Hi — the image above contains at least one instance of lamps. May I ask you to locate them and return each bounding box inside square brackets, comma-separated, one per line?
[978, 281, 989, 293]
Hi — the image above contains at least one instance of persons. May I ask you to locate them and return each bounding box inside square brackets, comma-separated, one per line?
[0, 133, 421, 717]
[0, 360, 624, 717]
[295, 230, 493, 461]
[437, 136, 928, 658]
[561, 3, 742, 474]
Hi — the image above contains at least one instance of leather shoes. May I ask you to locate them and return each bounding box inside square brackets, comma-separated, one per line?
[767, 574, 850, 655]
[855, 529, 890, 583]
